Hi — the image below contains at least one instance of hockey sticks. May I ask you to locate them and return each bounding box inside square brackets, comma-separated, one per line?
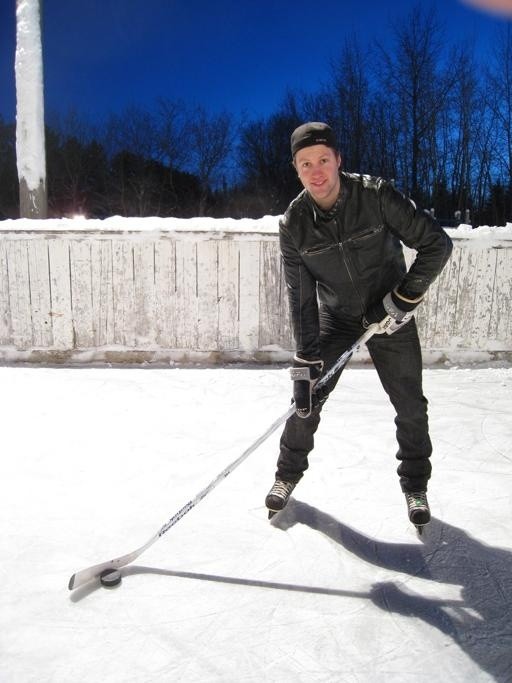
[68, 324, 379, 592]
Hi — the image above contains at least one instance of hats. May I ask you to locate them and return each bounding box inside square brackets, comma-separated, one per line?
[291, 121, 336, 157]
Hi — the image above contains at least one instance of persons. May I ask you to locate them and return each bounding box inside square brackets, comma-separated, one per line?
[264, 120, 454, 525]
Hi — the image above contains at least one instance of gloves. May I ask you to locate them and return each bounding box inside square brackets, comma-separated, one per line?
[365, 283, 424, 325]
[290, 353, 329, 418]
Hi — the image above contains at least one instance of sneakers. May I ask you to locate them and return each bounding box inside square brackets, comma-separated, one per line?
[406, 491, 430, 526]
[264, 480, 296, 509]
